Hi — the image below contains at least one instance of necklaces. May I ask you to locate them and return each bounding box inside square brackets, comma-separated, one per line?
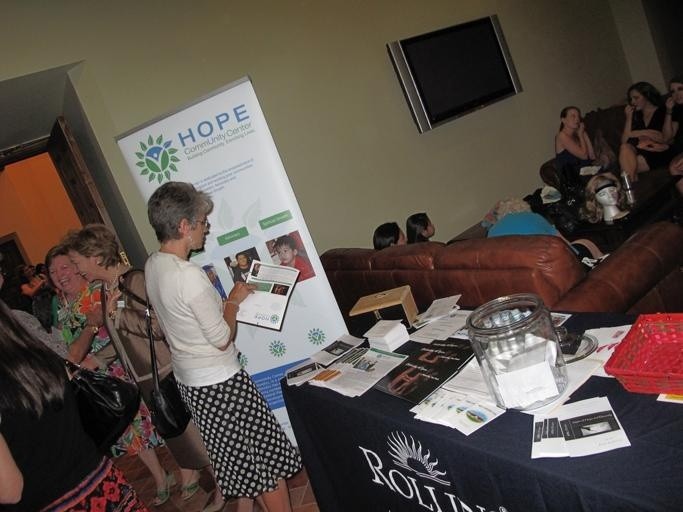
[106, 263, 120, 290]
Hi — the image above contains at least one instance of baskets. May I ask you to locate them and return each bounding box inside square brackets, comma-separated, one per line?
[604, 313, 683, 395]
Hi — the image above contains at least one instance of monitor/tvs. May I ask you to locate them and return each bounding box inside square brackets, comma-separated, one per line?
[386, 12, 524, 135]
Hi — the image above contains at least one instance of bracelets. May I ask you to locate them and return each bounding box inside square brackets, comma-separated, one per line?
[225, 300, 240, 305]
[87, 326, 98, 333]
[666, 108, 673, 115]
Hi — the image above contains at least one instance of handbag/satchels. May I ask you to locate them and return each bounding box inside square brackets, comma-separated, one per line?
[52, 348, 140, 450]
[144, 286, 191, 439]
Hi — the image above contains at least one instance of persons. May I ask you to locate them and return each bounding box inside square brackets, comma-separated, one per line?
[273, 236, 312, 283]
[45, 243, 201, 505]
[661, 76, 683, 195]
[144, 181, 303, 512]
[12, 264, 57, 334]
[373, 222, 405, 250]
[406, 213, 435, 244]
[554, 106, 608, 187]
[60, 226, 224, 511]
[231, 251, 251, 282]
[618, 82, 669, 183]
[483, 197, 602, 269]
[1, 303, 140, 511]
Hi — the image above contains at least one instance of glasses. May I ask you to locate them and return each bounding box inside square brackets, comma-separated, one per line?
[194, 219, 208, 227]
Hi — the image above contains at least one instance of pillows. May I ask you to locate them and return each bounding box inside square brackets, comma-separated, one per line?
[319, 234, 588, 298]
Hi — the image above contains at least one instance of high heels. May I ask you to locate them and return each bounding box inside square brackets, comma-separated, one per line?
[179, 480, 199, 501]
[152, 469, 177, 506]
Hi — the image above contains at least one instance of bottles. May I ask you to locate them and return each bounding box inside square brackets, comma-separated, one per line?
[625, 190, 637, 206]
[621, 170, 633, 190]
[464, 293, 570, 413]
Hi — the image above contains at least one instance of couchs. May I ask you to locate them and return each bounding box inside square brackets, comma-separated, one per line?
[320, 220, 683, 314]
[540, 92, 683, 202]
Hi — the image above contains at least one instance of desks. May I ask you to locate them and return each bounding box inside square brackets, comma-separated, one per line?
[280, 303, 683, 512]
